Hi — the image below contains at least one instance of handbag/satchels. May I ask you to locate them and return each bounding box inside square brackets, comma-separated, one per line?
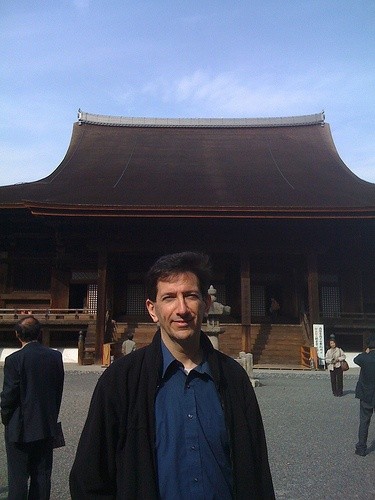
[341, 361, 349, 370]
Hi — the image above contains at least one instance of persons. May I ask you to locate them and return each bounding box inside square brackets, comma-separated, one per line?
[0, 315, 64, 500]
[69, 252, 277, 500]
[353, 336, 375, 456]
[325, 338, 347, 397]
[121, 333, 137, 355]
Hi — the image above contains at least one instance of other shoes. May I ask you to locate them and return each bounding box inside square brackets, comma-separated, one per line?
[354, 450, 366, 456]
